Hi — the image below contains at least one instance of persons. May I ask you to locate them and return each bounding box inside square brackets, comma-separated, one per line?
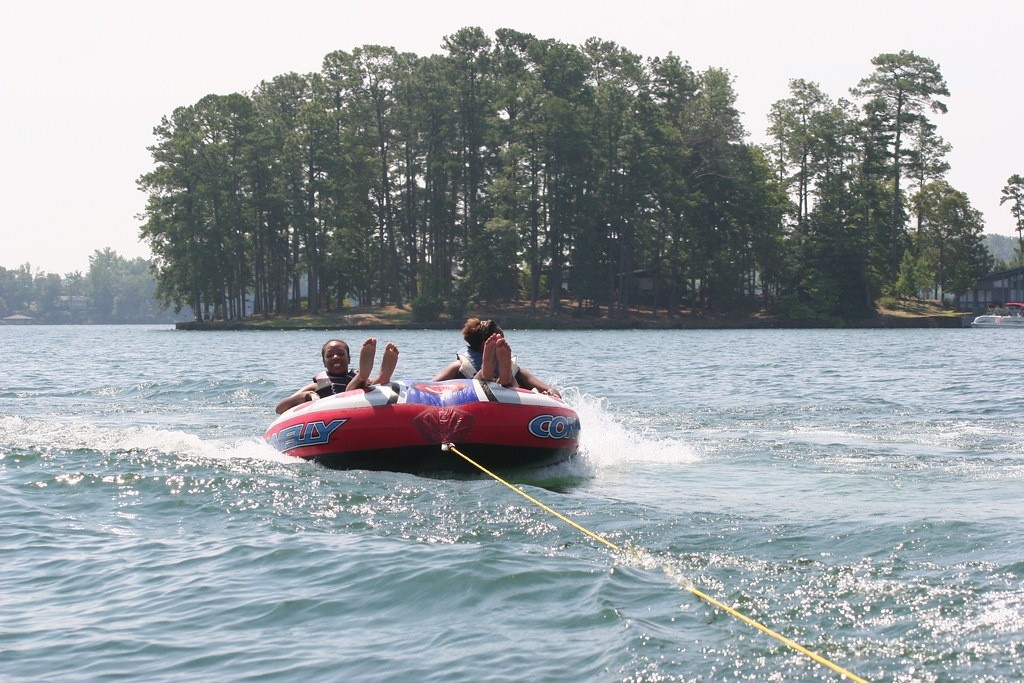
[429, 316, 562, 400]
[274, 337, 399, 415]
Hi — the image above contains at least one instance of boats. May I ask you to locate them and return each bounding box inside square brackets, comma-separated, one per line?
[970, 301, 1024, 329]
